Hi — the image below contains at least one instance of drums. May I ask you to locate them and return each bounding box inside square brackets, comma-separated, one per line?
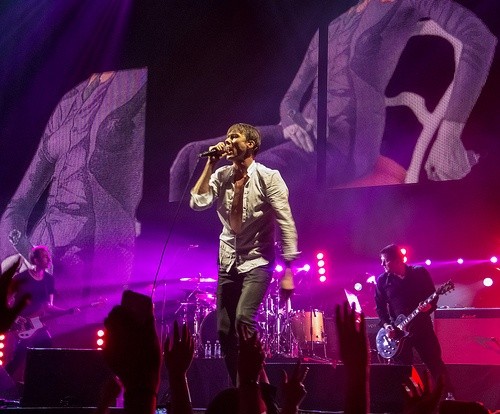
[199, 311, 217, 348]
[291, 311, 327, 349]
[184, 290, 213, 309]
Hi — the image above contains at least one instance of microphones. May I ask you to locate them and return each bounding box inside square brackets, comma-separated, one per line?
[198, 146, 229, 157]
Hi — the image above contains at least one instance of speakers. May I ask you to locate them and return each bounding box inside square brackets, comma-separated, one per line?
[17, 346, 114, 409]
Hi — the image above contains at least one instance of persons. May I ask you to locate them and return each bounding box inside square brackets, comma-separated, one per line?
[375, 244, 450, 402]
[95, 301, 371, 414]
[189, 123, 301, 387]
[4, 246, 82, 375]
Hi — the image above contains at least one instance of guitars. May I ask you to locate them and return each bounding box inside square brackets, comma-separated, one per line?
[13, 297, 108, 342]
[376, 276, 459, 358]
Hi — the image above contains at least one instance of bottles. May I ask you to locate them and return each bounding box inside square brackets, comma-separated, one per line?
[446, 392, 455, 401]
[214, 340, 220, 359]
[156, 408, 167, 414]
[192, 334, 198, 357]
[204, 341, 211, 359]
[292, 339, 299, 358]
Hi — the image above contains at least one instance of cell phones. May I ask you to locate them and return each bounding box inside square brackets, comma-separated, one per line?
[122, 290, 152, 322]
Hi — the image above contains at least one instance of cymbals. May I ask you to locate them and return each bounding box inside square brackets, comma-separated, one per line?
[180, 277, 218, 282]
